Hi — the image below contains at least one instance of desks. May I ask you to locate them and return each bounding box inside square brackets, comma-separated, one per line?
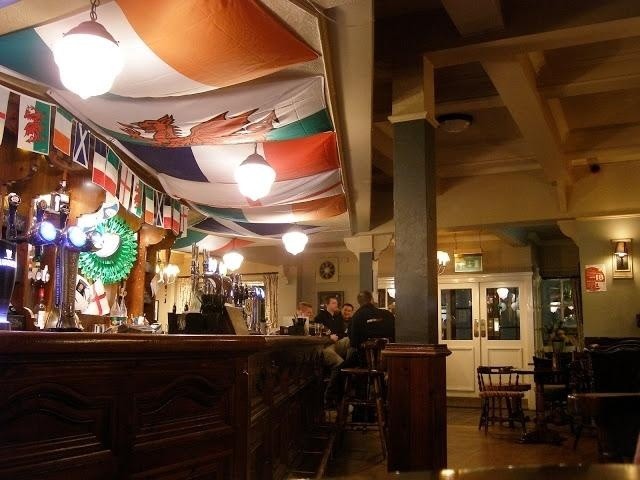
[568, 392, 640, 460]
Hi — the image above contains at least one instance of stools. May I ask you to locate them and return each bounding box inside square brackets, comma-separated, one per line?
[340, 366, 389, 461]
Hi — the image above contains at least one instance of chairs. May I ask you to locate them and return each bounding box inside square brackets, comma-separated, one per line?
[476, 365, 531, 435]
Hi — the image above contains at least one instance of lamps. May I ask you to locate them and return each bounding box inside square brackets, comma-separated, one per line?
[234, 142, 276, 202]
[282, 222, 308, 256]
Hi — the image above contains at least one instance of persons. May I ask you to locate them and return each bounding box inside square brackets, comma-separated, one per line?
[340, 303, 353, 329]
[296, 301, 313, 322]
[342, 289, 395, 423]
[314, 295, 352, 411]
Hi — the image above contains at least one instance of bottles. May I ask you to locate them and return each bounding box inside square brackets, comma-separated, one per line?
[314, 322, 323, 337]
[32, 287, 46, 330]
[109, 295, 129, 325]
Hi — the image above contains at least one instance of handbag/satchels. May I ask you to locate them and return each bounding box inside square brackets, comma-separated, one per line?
[566, 350, 594, 391]
